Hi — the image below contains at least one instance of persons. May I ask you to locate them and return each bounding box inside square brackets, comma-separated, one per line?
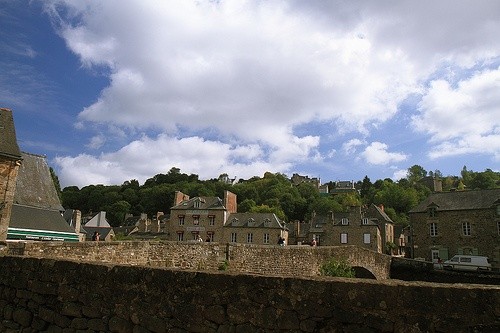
[196, 235, 203, 242]
[438, 257, 443, 269]
[92, 232, 100, 241]
[277, 237, 286, 246]
[311, 238, 316, 248]
[206, 235, 210, 242]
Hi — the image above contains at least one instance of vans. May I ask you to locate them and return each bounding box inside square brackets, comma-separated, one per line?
[442, 254, 492, 272]
